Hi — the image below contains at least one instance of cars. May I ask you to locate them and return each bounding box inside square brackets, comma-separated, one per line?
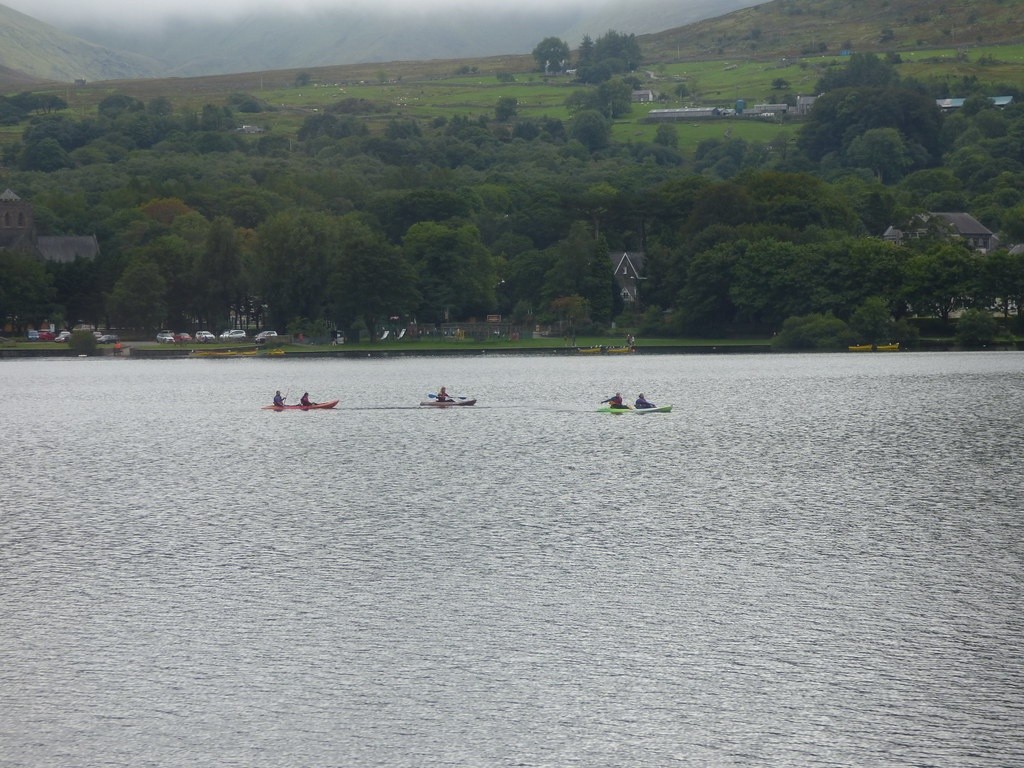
[255, 332, 271, 344]
[55, 334, 71, 342]
[219, 330, 246, 341]
[174, 333, 192, 342]
[39, 333, 56, 342]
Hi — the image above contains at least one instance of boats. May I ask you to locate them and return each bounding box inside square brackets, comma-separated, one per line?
[876, 343, 899, 349]
[849, 345, 872, 349]
[606, 347, 635, 355]
[420, 397, 477, 407]
[262, 398, 337, 410]
[579, 347, 606, 354]
[596, 403, 674, 415]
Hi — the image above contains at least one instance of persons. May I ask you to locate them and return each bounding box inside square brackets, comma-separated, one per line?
[636, 393, 657, 409]
[274, 390, 286, 407]
[436, 387, 454, 402]
[601, 392, 629, 408]
[113, 340, 122, 356]
[301, 392, 319, 406]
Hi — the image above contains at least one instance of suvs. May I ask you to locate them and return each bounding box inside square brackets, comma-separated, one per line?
[97, 335, 120, 344]
[255, 331, 277, 337]
[60, 332, 70, 336]
[157, 334, 173, 344]
[194, 331, 215, 341]
[157, 330, 174, 337]
[94, 332, 102, 338]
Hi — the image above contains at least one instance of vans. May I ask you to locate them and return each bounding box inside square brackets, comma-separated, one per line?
[26, 330, 40, 341]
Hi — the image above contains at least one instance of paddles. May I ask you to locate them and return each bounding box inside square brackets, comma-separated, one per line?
[428, 393, 467, 399]
[283, 388, 290, 405]
